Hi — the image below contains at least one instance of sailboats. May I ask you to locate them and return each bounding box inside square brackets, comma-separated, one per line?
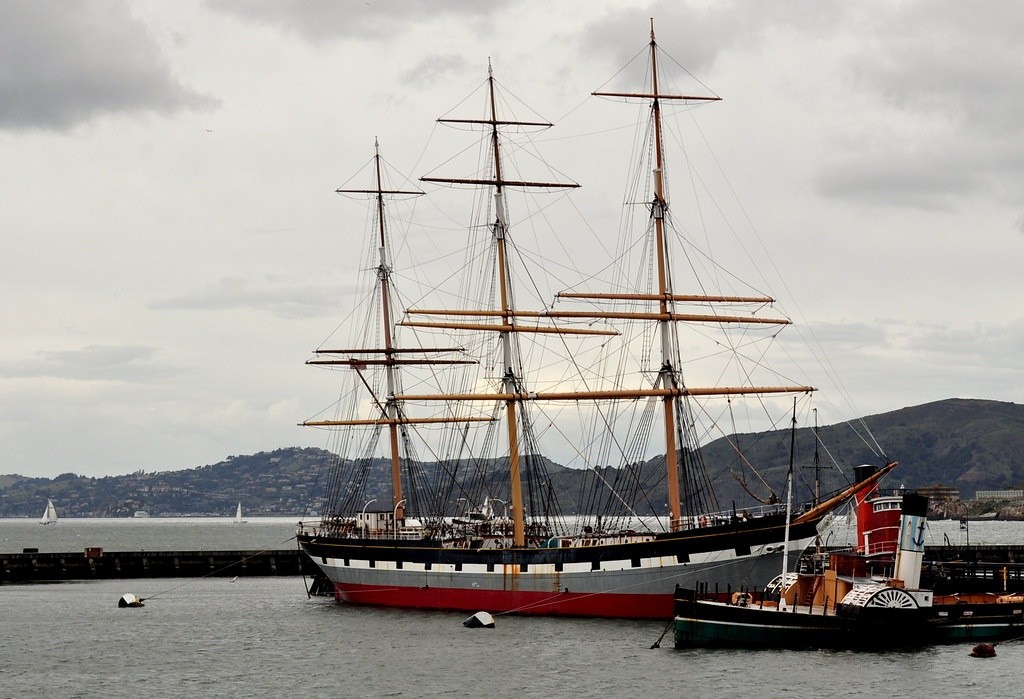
[39, 499, 59, 529]
[232, 501, 248, 524]
[287, 9, 902, 625]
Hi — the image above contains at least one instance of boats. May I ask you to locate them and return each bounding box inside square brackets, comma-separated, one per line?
[648, 462, 1024, 655]
